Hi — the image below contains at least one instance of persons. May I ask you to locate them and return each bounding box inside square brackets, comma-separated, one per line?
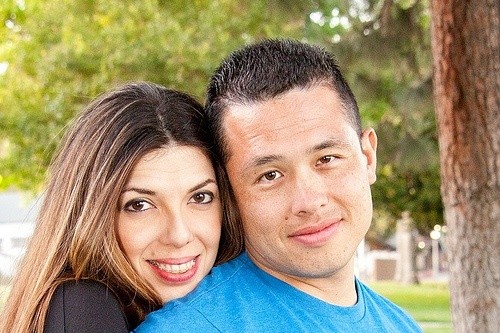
[0, 78, 245, 333]
[126, 36, 422, 333]
